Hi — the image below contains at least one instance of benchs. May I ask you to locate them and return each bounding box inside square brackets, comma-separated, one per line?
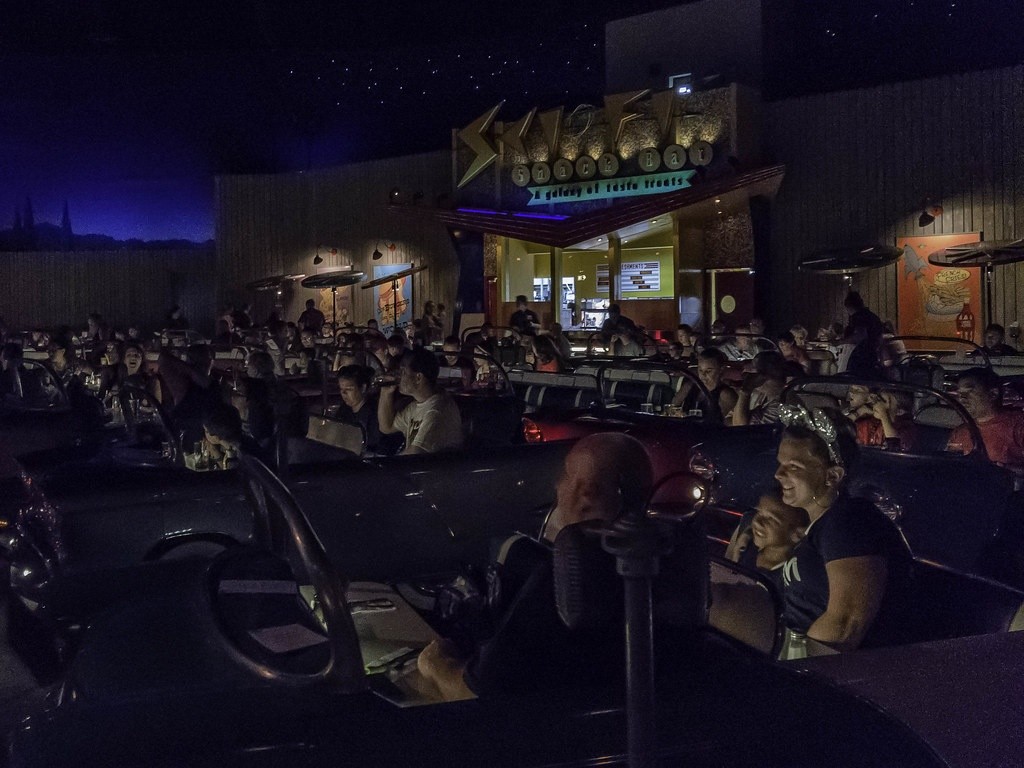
[1, 323, 1024, 700]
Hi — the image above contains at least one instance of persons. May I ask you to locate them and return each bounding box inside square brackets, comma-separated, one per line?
[0, 290, 1024, 702]
[945, 367, 1024, 477]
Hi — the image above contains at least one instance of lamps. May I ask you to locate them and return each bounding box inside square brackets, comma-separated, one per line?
[315, 243, 337, 265]
[372, 238, 396, 261]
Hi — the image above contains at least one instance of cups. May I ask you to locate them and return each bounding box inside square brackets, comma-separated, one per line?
[371, 374, 400, 388]
[689, 409, 702, 416]
[641, 403, 653, 413]
